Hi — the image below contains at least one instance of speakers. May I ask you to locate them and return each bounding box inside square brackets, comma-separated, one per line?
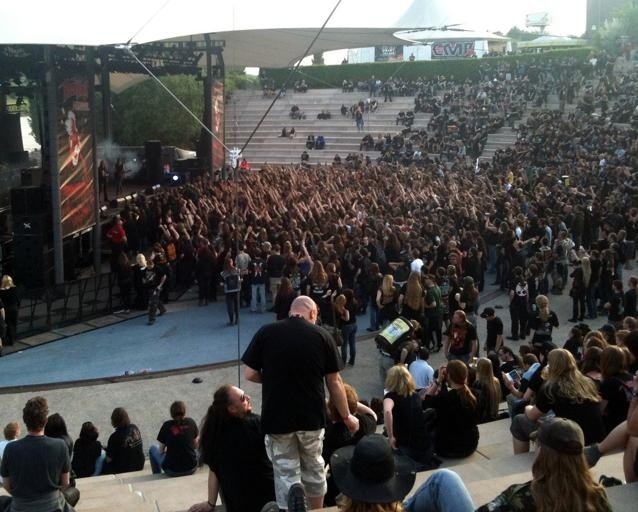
[100, 205, 108, 215]
[131, 192, 138, 199]
[146, 184, 160, 193]
[110, 197, 125, 208]
[124, 194, 132, 204]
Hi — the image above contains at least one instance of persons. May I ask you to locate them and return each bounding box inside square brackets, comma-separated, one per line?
[2, 44, 635, 512]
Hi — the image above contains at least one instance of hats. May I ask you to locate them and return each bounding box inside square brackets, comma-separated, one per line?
[330, 432, 416, 503]
[534, 342, 557, 355]
[464, 276, 472, 283]
[480, 308, 495, 318]
[529, 417, 585, 454]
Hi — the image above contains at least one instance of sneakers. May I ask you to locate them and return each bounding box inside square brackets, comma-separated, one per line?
[506, 335, 525, 341]
[287, 484, 308, 512]
[567, 317, 583, 322]
[158, 309, 166, 316]
[366, 328, 378, 331]
[262, 502, 279, 512]
[582, 444, 600, 467]
[226, 321, 238, 326]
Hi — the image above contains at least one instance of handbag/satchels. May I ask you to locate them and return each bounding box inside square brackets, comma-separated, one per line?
[322, 326, 342, 346]
